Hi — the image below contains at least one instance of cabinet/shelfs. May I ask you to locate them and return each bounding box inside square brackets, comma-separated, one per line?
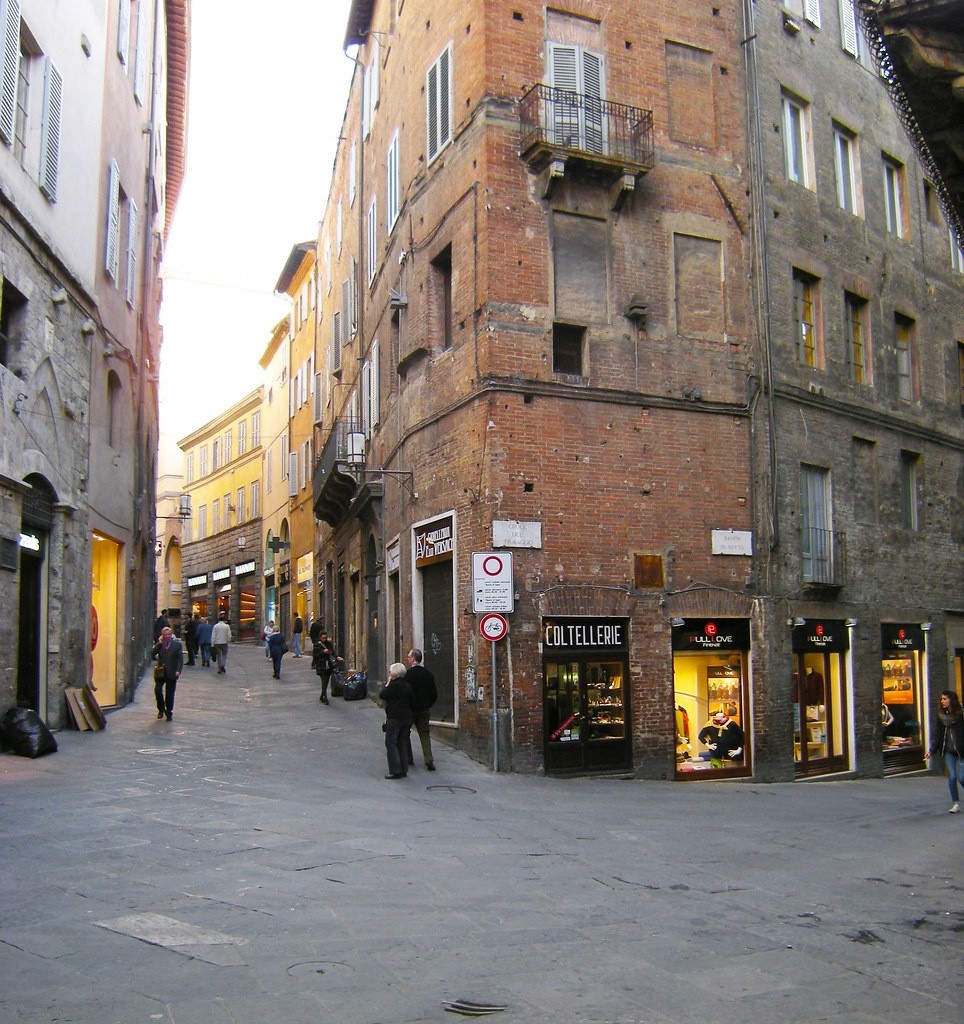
[586, 686, 623, 737]
[794, 720, 825, 759]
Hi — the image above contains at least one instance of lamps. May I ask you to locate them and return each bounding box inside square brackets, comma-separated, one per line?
[346, 432, 414, 501]
[921, 622, 933, 630]
[672, 617, 685, 627]
[845, 618, 859, 627]
[794, 616, 806, 626]
[149, 494, 192, 535]
[238, 535, 264, 560]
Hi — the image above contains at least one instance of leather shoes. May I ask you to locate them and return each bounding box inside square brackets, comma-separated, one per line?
[166, 713, 173, 721]
[157, 711, 164, 719]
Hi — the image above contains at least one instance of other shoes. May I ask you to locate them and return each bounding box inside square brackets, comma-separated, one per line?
[383, 772, 407, 779]
[425, 761, 435, 771]
[217, 667, 226, 675]
[202, 662, 205, 666]
[265, 657, 269, 661]
[275, 673, 280, 679]
[320, 694, 328, 704]
[207, 661, 210, 667]
[183, 661, 195, 666]
[273, 671, 275, 677]
[292, 655, 303, 658]
[269, 658, 272, 662]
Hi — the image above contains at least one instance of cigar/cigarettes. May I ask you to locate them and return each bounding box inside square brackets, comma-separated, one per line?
[923, 758, 927, 761]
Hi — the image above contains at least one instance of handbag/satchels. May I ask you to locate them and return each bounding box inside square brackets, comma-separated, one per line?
[261, 629, 267, 639]
[328, 655, 339, 669]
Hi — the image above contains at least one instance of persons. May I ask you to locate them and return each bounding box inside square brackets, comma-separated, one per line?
[151, 609, 170, 661]
[151, 627, 183, 721]
[196, 617, 211, 667]
[698, 713, 744, 760]
[183, 612, 196, 666]
[264, 621, 274, 661]
[675, 705, 692, 758]
[310, 617, 325, 669]
[379, 663, 414, 780]
[924, 691, 964, 812]
[194, 615, 200, 656]
[269, 626, 283, 679]
[210, 618, 231, 674]
[405, 648, 438, 771]
[292, 612, 303, 658]
[313, 630, 336, 705]
[882, 703, 893, 727]
[805, 667, 824, 705]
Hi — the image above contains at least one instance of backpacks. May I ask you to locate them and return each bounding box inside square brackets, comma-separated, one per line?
[281, 636, 288, 654]
